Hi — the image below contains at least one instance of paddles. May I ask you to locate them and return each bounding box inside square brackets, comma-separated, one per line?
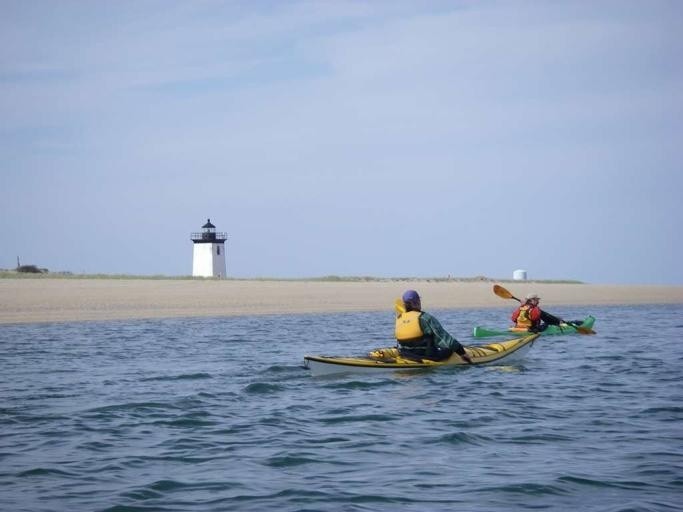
[394, 298, 406, 316]
[493, 284, 596, 334]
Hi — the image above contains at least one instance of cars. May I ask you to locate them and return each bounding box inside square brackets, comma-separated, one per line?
[18, 263, 48, 275]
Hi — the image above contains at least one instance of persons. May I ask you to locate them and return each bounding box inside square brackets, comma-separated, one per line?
[512, 293, 564, 331]
[395, 290, 472, 365]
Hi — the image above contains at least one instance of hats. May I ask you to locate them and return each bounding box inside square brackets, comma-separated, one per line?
[402, 290, 420, 303]
[526, 292, 541, 300]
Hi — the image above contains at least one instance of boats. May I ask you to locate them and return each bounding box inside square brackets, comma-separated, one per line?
[297, 330, 543, 378]
[472, 317, 598, 343]
